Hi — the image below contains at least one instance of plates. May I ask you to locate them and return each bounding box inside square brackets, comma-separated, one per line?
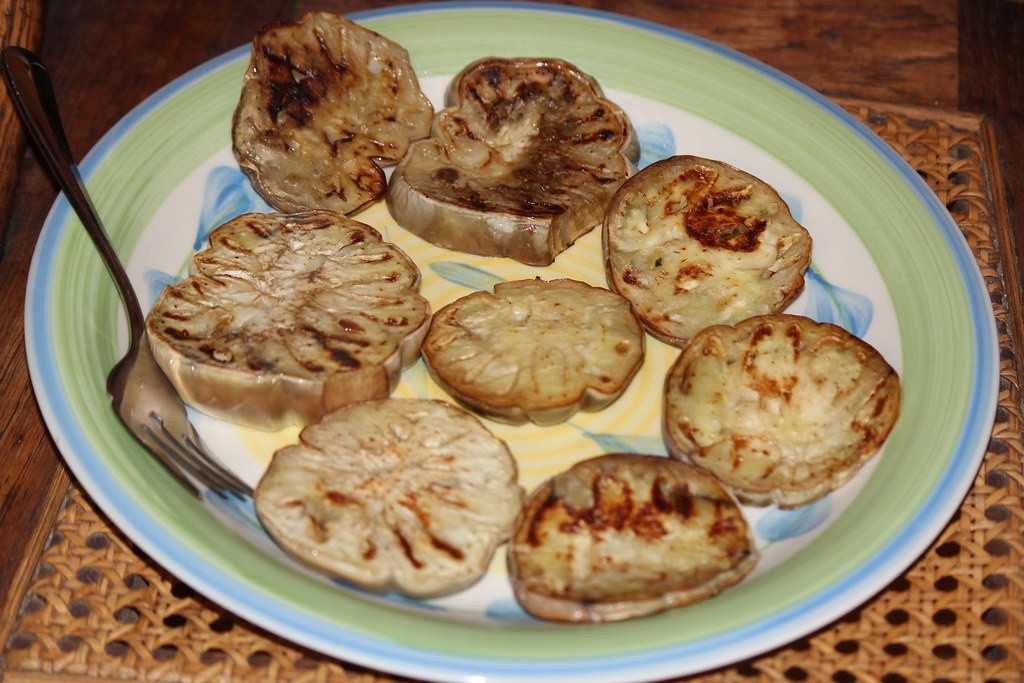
[23, 1, 999, 683]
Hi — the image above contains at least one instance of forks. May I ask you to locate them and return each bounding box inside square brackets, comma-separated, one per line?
[0, 47, 256, 502]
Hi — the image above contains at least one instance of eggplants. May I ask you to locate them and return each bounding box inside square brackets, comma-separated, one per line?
[140, 7, 902, 625]
